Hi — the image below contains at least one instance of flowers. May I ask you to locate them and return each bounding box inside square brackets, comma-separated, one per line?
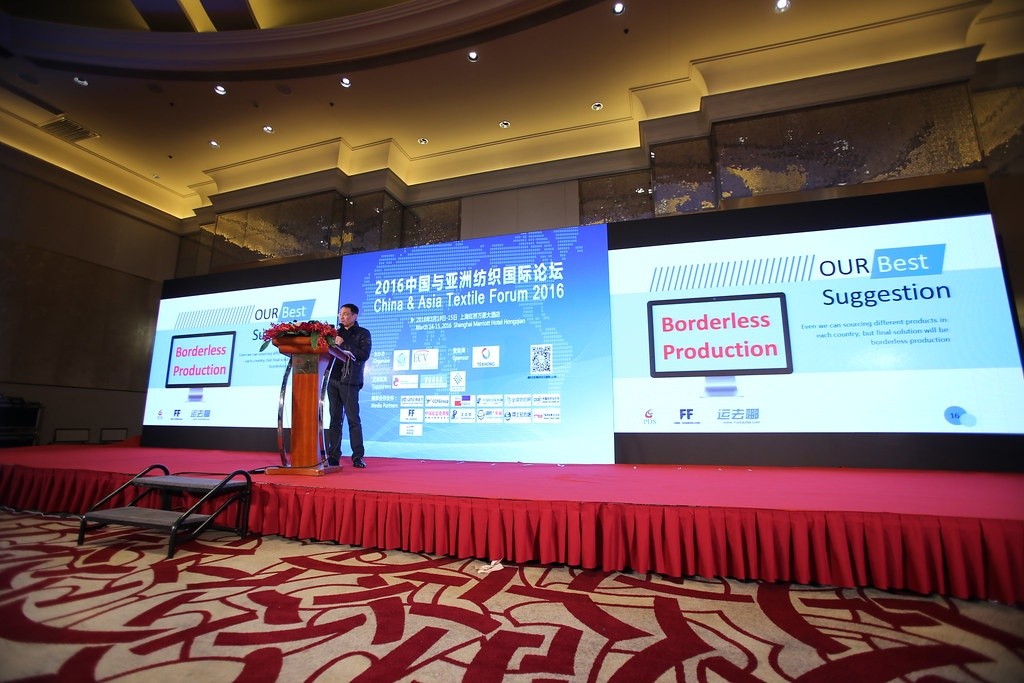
[259, 320, 339, 352]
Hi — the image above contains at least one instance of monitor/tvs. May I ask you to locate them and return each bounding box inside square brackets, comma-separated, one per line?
[648, 292, 792, 397]
[165, 331, 236, 402]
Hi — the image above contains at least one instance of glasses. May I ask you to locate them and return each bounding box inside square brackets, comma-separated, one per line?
[339, 313, 351, 316]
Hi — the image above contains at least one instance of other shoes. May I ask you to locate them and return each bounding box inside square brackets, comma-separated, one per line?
[353, 457, 366, 467]
[328, 458, 339, 466]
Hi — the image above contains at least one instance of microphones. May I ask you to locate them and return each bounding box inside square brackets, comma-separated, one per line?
[337, 323, 344, 337]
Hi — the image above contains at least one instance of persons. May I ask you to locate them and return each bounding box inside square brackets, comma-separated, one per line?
[327, 304, 371, 468]
